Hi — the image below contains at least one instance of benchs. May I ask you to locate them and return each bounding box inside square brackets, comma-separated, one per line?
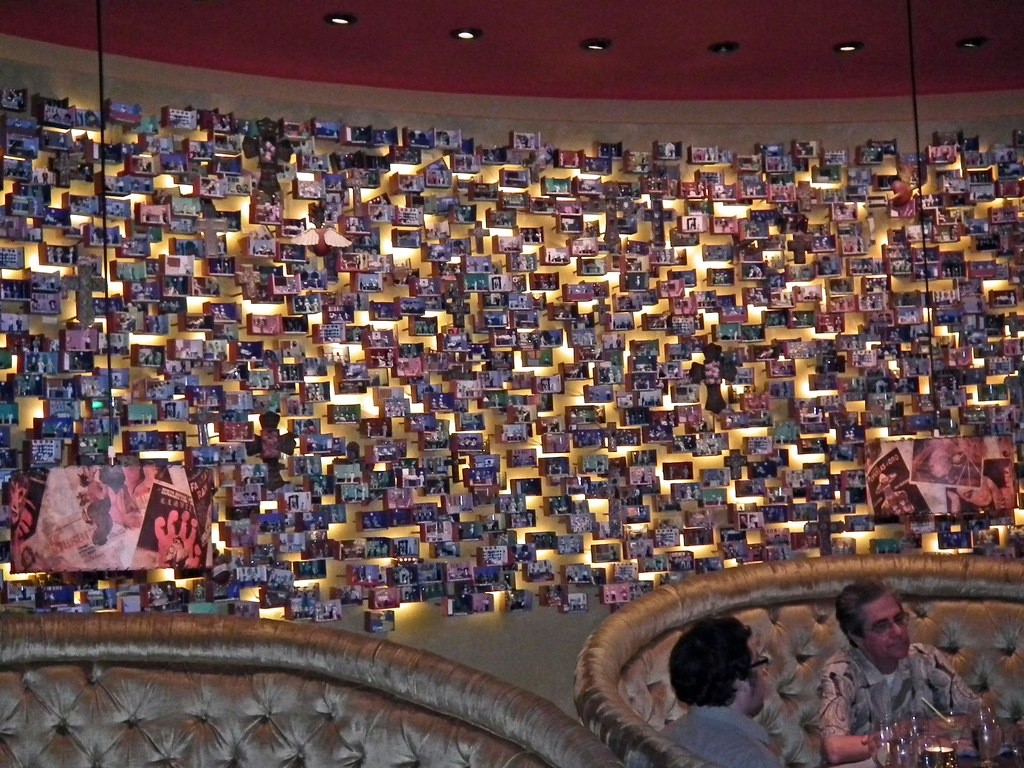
[0, 609, 624, 767]
[571, 552, 1024, 768]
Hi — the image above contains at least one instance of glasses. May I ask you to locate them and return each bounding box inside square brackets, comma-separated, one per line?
[862, 611, 910, 634]
[750, 655, 768, 669]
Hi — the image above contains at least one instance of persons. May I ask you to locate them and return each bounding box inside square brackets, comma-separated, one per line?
[1, 87, 1024, 632]
[816, 579, 1002, 768]
[659, 616, 780, 768]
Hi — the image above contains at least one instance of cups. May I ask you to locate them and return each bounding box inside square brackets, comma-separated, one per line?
[867, 706, 943, 768]
[936, 704, 1024, 768]
[919, 746, 958, 768]
